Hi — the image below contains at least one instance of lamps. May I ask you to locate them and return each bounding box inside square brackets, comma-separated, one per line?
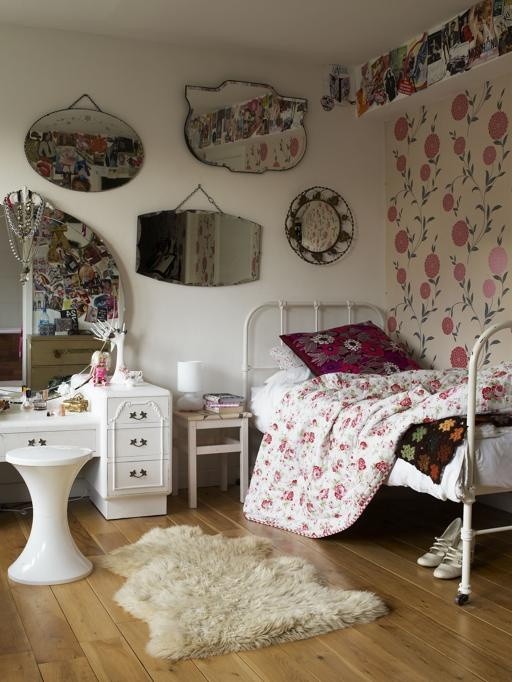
[176, 361, 204, 411]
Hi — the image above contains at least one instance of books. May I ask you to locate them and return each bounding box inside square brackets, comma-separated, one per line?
[203, 392, 245, 404]
[204, 404, 244, 414]
[206, 400, 240, 408]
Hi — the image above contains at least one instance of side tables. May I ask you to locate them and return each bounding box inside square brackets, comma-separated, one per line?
[173, 410, 252, 509]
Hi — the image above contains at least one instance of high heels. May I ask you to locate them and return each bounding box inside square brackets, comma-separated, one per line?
[416, 517, 475, 579]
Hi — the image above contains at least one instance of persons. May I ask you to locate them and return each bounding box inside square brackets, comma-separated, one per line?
[25, 131, 143, 192]
[34, 210, 121, 323]
[438, 0, 496, 64]
[90, 351, 111, 386]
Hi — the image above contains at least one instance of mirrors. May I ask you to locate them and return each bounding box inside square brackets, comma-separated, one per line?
[135, 209, 261, 286]
[0, 205, 125, 404]
[184, 80, 308, 173]
[24, 108, 145, 192]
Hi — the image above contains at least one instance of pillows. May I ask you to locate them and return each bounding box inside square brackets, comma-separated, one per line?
[264, 367, 311, 384]
[279, 321, 424, 377]
[270, 343, 307, 374]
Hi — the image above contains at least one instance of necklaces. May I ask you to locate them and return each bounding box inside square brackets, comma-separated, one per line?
[3, 189, 47, 284]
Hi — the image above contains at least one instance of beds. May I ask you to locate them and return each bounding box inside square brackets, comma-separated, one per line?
[241, 300, 511, 605]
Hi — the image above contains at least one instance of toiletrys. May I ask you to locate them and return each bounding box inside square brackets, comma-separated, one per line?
[33, 394, 47, 410]
[19, 390, 33, 411]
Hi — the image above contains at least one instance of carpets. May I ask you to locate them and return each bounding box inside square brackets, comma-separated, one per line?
[87, 525, 389, 660]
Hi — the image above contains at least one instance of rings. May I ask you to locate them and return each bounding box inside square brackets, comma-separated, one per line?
[106, 326, 128, 341]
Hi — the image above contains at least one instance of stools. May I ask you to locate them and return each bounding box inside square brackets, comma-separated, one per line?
[5, 445, 94, 586]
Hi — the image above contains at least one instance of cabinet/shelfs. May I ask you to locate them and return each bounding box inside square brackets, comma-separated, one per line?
[26, 334, 108, 391]
[0, 381, 173, 520]
[0, 333, 22, 380]
